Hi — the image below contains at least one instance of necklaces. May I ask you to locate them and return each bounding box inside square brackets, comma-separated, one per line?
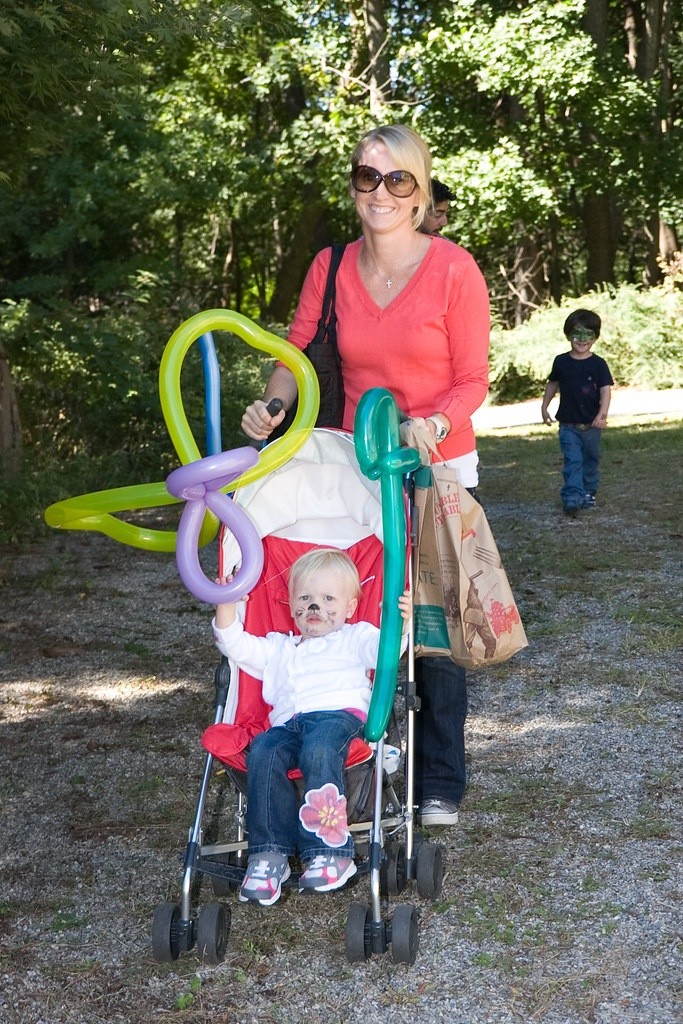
[364, 244, 412, 289]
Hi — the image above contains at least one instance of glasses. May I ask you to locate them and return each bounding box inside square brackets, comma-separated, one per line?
[349, 165, 419, 199]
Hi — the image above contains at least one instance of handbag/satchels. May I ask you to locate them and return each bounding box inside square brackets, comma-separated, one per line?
[411, 465, 529, 668]
[267, 343, 345, 443]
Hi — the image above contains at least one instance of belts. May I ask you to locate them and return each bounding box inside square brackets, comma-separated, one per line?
[560, 420, 593, 430]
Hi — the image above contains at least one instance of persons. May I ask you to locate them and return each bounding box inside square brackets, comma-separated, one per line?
[413, 177, 457, 238]
[239, 125, 492, 828]
[212, 549, 414, 912]
[541, 308, 615, 514]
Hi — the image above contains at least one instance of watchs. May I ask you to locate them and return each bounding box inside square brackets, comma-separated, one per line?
[426, 416, 447, 445]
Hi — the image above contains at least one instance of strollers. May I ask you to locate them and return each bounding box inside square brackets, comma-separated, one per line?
[148, 397, 446, 969]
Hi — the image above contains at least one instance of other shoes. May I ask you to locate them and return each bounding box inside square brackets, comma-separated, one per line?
[584, 492, 595, 508]
[565, 504, 581, 520]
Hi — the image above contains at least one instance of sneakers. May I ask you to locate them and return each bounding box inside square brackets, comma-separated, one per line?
[297, 856, 356, 892]
[237, 853, 292, 905]
[422, 799, 459, 827]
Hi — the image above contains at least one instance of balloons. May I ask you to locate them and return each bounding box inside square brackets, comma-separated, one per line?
[45, 309, 321, 602]
[355, 387, 422, 742]
